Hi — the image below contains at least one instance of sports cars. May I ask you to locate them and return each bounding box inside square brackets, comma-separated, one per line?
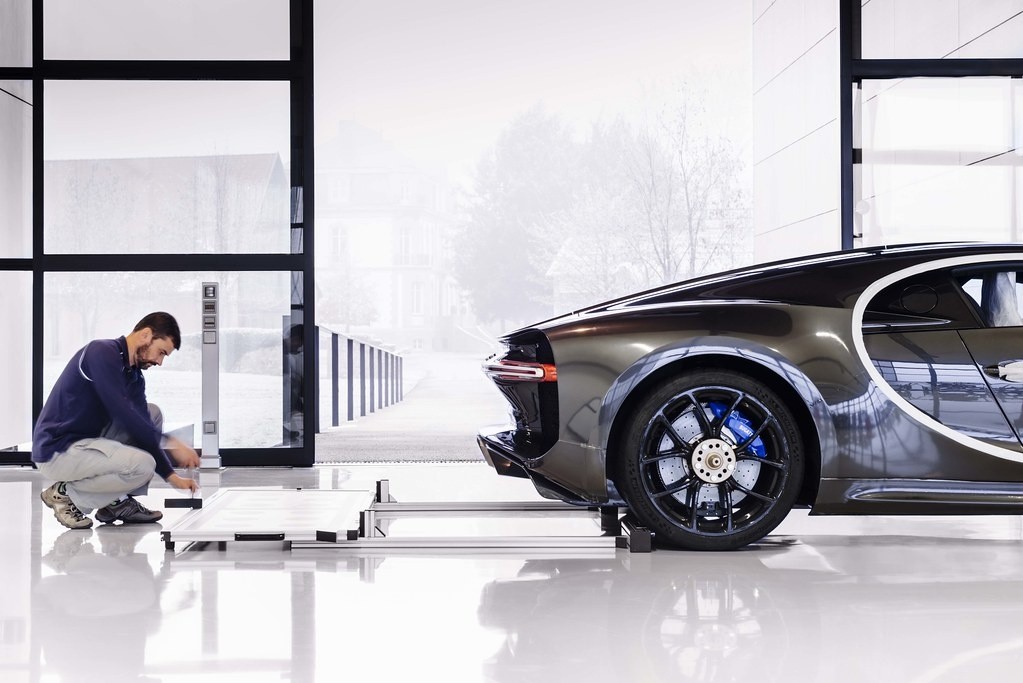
[477, 239, 1023, 551]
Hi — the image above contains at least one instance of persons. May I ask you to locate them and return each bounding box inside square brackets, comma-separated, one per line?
[31, 311, 201, 529]
[32, 523, 163, 683]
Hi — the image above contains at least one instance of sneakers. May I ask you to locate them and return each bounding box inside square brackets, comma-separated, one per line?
[41, 482, 93, 529]
[95, 496, 163, 525]
